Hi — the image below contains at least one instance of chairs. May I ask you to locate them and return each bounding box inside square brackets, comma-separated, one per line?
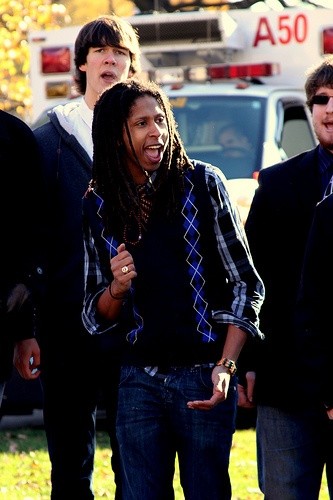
[281, 120, 314, 160]
[190, 109, 233, 148]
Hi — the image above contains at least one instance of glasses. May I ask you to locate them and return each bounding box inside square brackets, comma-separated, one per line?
[311, 95, 333, 105]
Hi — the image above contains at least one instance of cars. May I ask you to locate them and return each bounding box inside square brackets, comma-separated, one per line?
[135, 65, 318, 232]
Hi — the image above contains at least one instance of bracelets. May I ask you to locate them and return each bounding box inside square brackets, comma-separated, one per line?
[216, 359, 238, 375]
[108, 283, 125, 300]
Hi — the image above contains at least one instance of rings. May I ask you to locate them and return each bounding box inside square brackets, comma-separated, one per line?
[122, 266, 129, 273]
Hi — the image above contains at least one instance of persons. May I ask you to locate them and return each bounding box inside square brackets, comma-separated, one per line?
[216, 126, 254, 159]
[0, 108, 65, 410]
[79, 82, 267, 500]
[245, 56, 333, 500]
[14, 17, 139, 500]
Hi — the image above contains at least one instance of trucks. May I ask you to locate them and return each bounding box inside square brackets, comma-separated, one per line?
[29, 8, 333, 130]
